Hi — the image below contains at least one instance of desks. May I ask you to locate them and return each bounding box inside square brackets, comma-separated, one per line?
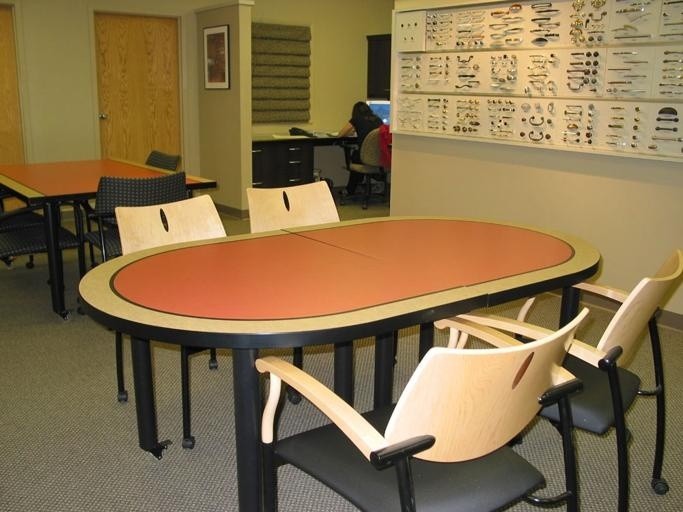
[0, 159, 217, 322]
[253, 136, 357, 188]
[79, 218, 601, 512]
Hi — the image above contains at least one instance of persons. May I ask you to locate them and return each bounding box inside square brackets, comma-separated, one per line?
[336, 101, 385, 195]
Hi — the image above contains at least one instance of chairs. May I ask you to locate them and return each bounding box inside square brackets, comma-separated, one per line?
[0, 199, 44, 232]
[256, 308, 588, 512]
[457, 249, 682, 512]
[341, 130, 392, 210]
[0, 188, 77, 290]
[246, 182, 397, 367]
[146, 150, 180, 170]
[114, 196, 303, 448]
[84, 169, 188, 268]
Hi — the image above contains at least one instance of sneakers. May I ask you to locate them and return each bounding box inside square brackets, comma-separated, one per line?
[338, 189, 348, 195]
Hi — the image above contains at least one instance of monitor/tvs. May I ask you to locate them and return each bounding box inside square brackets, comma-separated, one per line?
[366, 101, 390, 126]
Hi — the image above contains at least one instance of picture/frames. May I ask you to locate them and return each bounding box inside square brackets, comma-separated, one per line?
[205, 24, 230, 90]
